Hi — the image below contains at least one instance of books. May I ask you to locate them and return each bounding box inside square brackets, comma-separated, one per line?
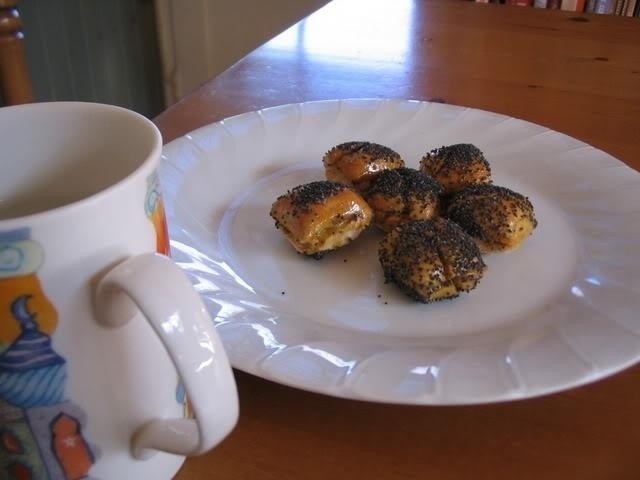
[468, 0, 639, 18]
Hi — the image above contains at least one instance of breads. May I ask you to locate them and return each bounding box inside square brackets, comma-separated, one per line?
[442, 182, 539, 253]
[419, 144, 491, 194]
[363, 166, 448, 234]
[323, 141, 404, 201]
[378, 214, 487, 305]
[269, 178, 375, 261]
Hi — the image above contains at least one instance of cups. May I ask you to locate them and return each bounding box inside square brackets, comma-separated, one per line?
[0, 99, 240, 480]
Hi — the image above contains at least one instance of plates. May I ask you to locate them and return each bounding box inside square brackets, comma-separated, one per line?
[153, 97, 640, 407]
